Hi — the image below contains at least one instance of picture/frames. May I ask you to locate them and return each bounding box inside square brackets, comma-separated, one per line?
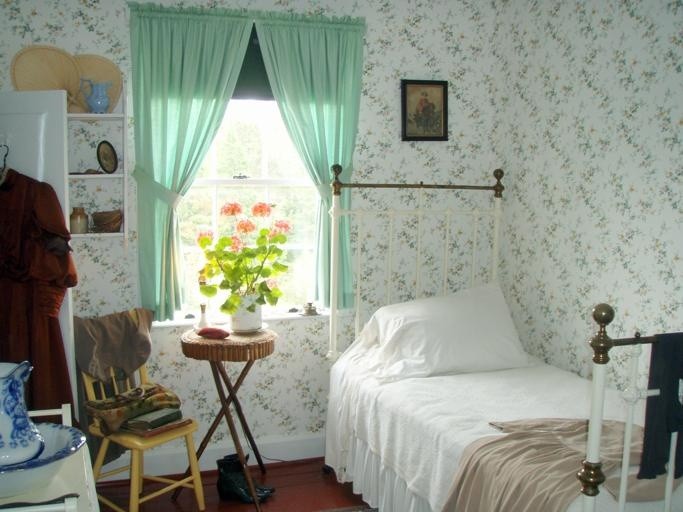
[0, 440, 99, 512]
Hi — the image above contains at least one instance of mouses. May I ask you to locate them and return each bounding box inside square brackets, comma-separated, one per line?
[0, 144, 9, 175]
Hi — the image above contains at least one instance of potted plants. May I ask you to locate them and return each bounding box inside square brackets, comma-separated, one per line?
[68, 90, 125, 237]
[401, 79, 447, 141]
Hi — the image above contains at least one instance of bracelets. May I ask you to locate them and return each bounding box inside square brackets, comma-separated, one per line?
[0, 419, 86, 498]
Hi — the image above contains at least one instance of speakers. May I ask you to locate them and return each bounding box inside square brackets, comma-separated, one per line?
[217, 454, 275, 503]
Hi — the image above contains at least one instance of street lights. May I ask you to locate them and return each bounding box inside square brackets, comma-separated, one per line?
[84, 382, 181, 433]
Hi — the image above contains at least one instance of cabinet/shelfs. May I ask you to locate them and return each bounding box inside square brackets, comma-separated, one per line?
[68, 206, 89, 235]
[197, 304, 209, 328]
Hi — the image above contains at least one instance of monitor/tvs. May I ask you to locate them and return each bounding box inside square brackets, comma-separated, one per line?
[120, 408, 182, 429]
[120, 417, 192, 437]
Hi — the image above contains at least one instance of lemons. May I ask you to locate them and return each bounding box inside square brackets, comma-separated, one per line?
[0, 359, 44, 465]
[79, 78, 113, 113]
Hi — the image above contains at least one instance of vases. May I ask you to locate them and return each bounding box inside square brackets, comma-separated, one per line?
[194, 201, 290, 315]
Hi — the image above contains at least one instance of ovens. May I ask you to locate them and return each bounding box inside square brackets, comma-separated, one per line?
[324, 162, 682, 512]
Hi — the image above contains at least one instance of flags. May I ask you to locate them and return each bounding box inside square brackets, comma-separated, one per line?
[231, 294, 262, 333]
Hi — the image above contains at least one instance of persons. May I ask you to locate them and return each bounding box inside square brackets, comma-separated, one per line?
[416, 91, 434, 116]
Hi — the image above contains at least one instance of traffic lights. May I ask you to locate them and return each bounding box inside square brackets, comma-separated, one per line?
[363, 286, 530, 387]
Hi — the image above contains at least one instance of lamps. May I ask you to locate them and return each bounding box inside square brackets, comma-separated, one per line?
[76, 310, 205, 511]
[27, 402, 73, 428]
